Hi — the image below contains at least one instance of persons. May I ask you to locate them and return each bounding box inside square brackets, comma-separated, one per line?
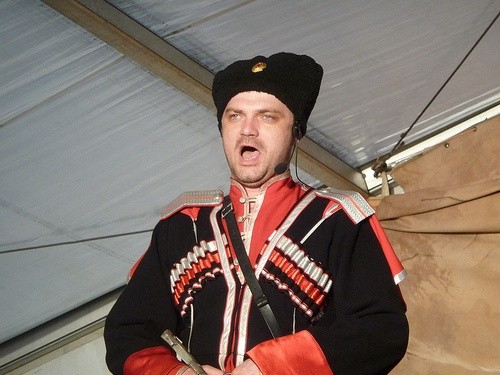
[104, 53, 410, 375]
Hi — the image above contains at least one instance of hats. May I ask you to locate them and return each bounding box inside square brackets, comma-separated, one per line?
[211, 51, 323, 135]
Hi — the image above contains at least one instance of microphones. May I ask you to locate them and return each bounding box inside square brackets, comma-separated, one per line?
[274, 138, 296, 174]
